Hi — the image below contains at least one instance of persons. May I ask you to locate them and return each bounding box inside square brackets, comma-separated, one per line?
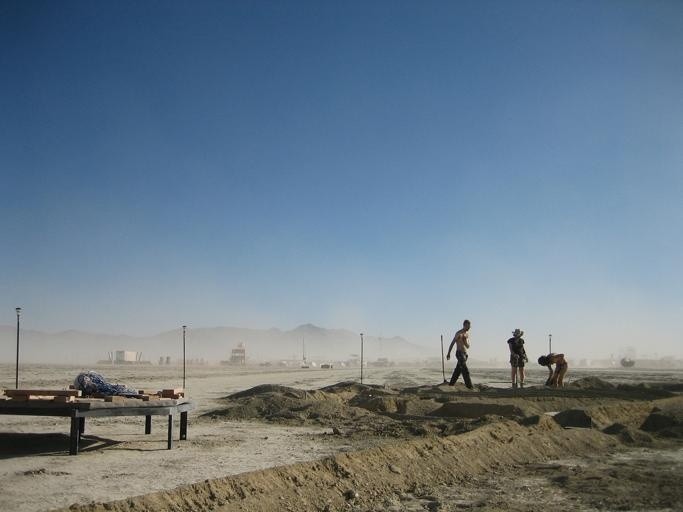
[445, 319, 474, 390]
[537, 352, 568, 387]
[505, 328, 528, 389]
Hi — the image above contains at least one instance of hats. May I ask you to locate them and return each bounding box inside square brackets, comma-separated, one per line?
[511, 329, 524, 336]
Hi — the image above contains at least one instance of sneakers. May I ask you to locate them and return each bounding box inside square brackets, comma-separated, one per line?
[512, 382, 524, 387]
